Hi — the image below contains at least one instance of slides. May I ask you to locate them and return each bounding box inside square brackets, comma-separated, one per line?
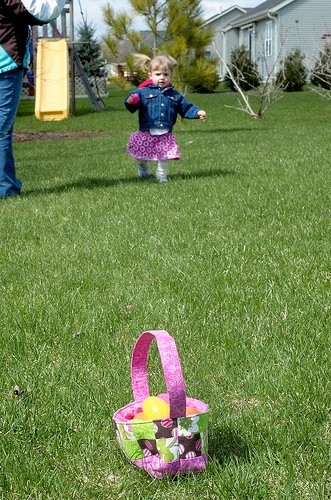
[34, 34, 70, 122]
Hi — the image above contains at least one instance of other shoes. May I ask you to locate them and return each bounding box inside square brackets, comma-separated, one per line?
[154, 172, 169, 183]
[138, 167, 150, 177]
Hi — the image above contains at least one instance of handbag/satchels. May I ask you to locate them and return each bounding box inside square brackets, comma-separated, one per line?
[110, 328, 215, 480]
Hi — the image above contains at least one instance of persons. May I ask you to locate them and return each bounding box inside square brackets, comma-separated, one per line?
[123, 53, 207, 184]
[0, 0, 66, 199]
